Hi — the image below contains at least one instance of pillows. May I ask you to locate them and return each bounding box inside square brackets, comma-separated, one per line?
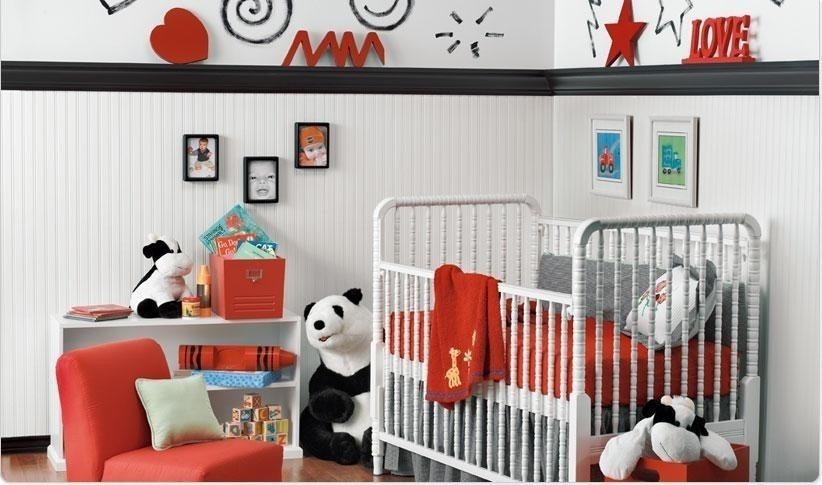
[135, 371, 225, 454]
[620, 255, 717, 348]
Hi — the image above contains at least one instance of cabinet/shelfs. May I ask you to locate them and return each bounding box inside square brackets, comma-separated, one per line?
[45, 306, 310, 472]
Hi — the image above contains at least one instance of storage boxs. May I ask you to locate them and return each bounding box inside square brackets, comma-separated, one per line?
[604, 443, 750, 482]
[210, 252, 288, 322]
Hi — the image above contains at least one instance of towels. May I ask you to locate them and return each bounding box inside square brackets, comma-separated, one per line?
[426, 264, 507, 410]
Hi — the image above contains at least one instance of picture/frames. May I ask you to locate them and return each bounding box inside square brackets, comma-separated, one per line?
[588, 114, 631, 199]
[647, 117, 699, 208]
[244, 156, 279, 203]
[294, 122, 328, 168]
[181, 132, 220, 183]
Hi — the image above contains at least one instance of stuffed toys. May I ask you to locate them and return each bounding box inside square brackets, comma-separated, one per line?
[298, 286, 392, 469]
[129, 232, 195, 320]
[598, 392, 738, 481]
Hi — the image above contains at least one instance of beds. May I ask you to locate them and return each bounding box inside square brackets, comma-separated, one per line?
[369, 193, 765, 480]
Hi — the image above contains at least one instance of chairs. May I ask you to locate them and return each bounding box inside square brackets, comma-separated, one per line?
[53, 337, 285, 482]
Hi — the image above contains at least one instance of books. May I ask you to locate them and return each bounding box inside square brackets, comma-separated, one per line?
[0, 433, 51, 454]
[198, 203, 283, 259]
[62, 302, 134, 323]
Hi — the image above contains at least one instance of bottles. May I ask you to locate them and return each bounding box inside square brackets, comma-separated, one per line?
[195, 265, 213, 317]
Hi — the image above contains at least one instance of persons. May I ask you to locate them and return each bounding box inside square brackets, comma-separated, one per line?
[187, 137, 216, 177]
[298, 126, 327, 166]
[248, 160, 275, 199]
